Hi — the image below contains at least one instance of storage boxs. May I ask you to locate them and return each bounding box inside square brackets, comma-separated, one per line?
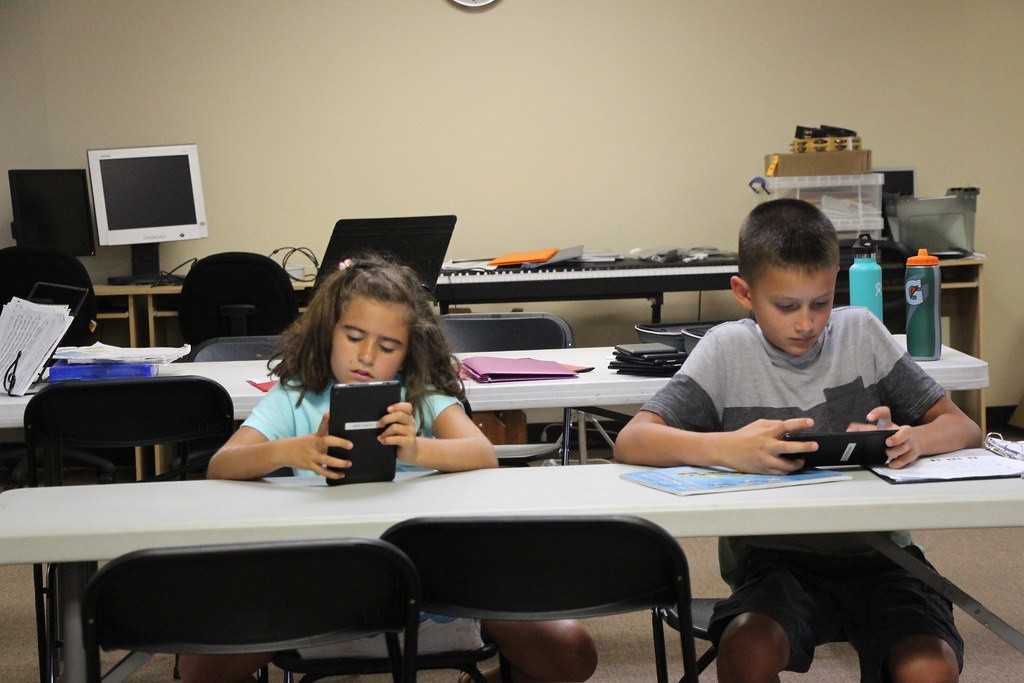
[750, 149, 884, 242]
[897, 195, 976, 255]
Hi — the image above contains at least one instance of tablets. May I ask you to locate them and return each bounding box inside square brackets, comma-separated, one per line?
[778, 429, 899, 467]
[325, 380, 401, 486]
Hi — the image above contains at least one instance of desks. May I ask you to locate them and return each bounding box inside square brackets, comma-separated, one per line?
[0, 256, 1024, 683]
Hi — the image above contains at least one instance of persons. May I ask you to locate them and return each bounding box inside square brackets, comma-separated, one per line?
[206, 257, 597, 683]
[614, 199, 983, 683]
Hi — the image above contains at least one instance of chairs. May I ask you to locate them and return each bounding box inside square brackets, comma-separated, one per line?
[0, 257, 99, 349]
[178, 250, 299, 351]
[428, 312, 573, 355]
[374, 514, 699, 683]
[652, 597, 780, 683]
[189, 335, 293, 371]
[27, 376, 234, 682]
[78, 537, 420, 683]
[266, 634, 496, 683]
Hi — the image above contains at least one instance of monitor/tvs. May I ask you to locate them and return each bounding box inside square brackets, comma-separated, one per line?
[8, 169, 96, 257]
[87, 143, 209, 285]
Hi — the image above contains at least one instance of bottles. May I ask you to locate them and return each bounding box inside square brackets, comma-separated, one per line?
[903, 249, 941, 361]
[849, 233, 883, 331]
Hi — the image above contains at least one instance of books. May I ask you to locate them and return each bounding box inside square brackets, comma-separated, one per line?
[620, 464, 852, 498]
